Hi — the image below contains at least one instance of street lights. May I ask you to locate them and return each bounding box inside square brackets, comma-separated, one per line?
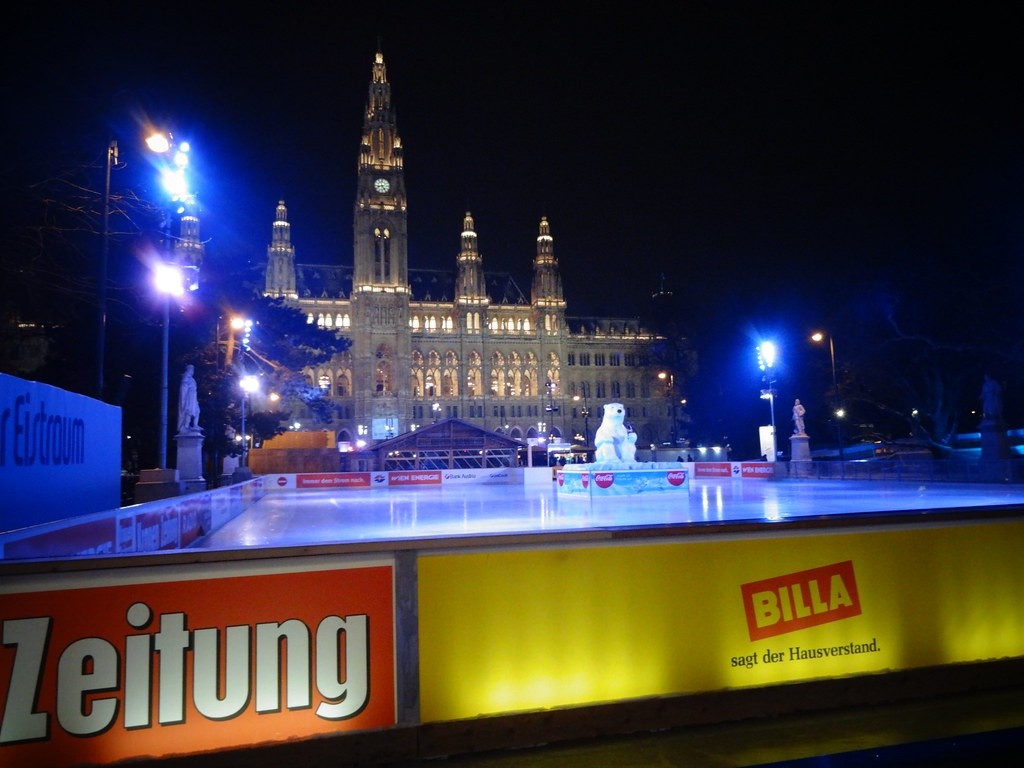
[150, 258, 182, 469]
[96, 125, 171, 400]
[213, 311, 248, 486]
[811, 330, 847, 480]
[572, 381, 590, 446]
[658, 369, 678, 449]
[432, 402, 442, 423]
[755, 339, 778, 462]
[544, 381, 559, 443]
[238, 375, 261, 465]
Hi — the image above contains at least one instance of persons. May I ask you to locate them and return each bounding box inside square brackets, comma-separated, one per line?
[177, 365, 204, 432]
[792, 399, 806, 434]
